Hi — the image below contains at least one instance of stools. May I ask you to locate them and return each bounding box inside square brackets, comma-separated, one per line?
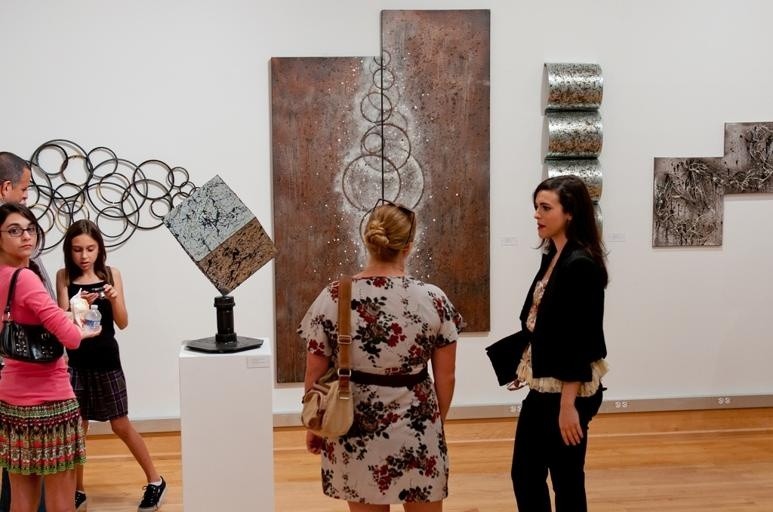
[350, 365, 428, 387]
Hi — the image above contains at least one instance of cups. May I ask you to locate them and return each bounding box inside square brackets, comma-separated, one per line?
[373, 199, 415, 244]
[0, 227, 40, 236]
[507, 381, 528, 390]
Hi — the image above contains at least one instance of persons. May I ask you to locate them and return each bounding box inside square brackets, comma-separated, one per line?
[295, 203, 468, 512]
[55, 219, 166, 512]
[0, 151, 57, 511]
[511, 175, 608, 512]
[0, 202, 83, 512]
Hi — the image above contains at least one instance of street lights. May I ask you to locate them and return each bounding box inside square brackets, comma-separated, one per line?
[302, 382, 354, 438]
[486, 331, 532, 386]
[0, 321, 64, 361]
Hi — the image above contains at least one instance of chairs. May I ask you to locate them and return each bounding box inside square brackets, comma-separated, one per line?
[139, 476, 167, 512]
[75, 491, 86, 511]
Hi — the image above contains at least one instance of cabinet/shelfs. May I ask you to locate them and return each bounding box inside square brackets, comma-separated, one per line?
[82, 304, 103, 333]
